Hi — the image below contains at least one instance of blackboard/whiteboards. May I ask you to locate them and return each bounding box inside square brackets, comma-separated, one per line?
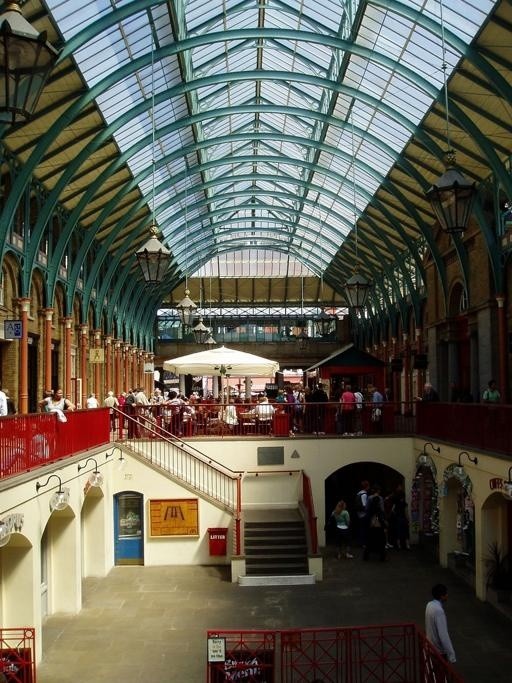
[210, 649, 274, 683]
[0, 648, 32, 683]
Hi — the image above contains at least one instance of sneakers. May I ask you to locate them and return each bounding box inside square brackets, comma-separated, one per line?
[292, 430, 363, 438]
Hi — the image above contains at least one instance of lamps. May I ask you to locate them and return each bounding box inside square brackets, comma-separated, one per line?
[36, 444, 130, 512]
[422, 1, 479, 234]
[295, 95, 373, 354]
[0, 0, 67, 127]
[135, 23, 228, 349]
[416, 442, 512, 501]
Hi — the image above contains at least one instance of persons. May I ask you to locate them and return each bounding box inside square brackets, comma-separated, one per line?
[330, 481, 411, 563]
[40, 389, 76, 412]
[84, 393, 99, 409]
[104, 391, 119, 432]
[483, 382, 500, 403]
[415, 383, 440, 401]
[0, 389, 16, 416]
[278, 383, 393, 436]
[423, 584, 457, 683]
[118, 388, 276, 439]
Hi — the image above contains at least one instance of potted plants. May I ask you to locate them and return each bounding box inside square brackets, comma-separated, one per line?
[480, 539, 511, 604]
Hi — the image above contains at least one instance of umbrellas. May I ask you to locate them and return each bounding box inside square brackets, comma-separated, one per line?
[163, 345, 280, 406]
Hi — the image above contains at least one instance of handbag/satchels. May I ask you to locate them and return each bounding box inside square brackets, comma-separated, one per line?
[324, 516, 337, 532]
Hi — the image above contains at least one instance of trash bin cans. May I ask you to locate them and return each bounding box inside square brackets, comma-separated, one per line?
[272, 414, 290, 437]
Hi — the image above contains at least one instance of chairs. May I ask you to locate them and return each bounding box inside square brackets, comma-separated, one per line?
[238, 411, 273, 435]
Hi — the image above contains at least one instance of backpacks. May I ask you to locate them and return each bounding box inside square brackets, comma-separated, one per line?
[355, 493, 367, 508]
[294, 392, 303, 418]
